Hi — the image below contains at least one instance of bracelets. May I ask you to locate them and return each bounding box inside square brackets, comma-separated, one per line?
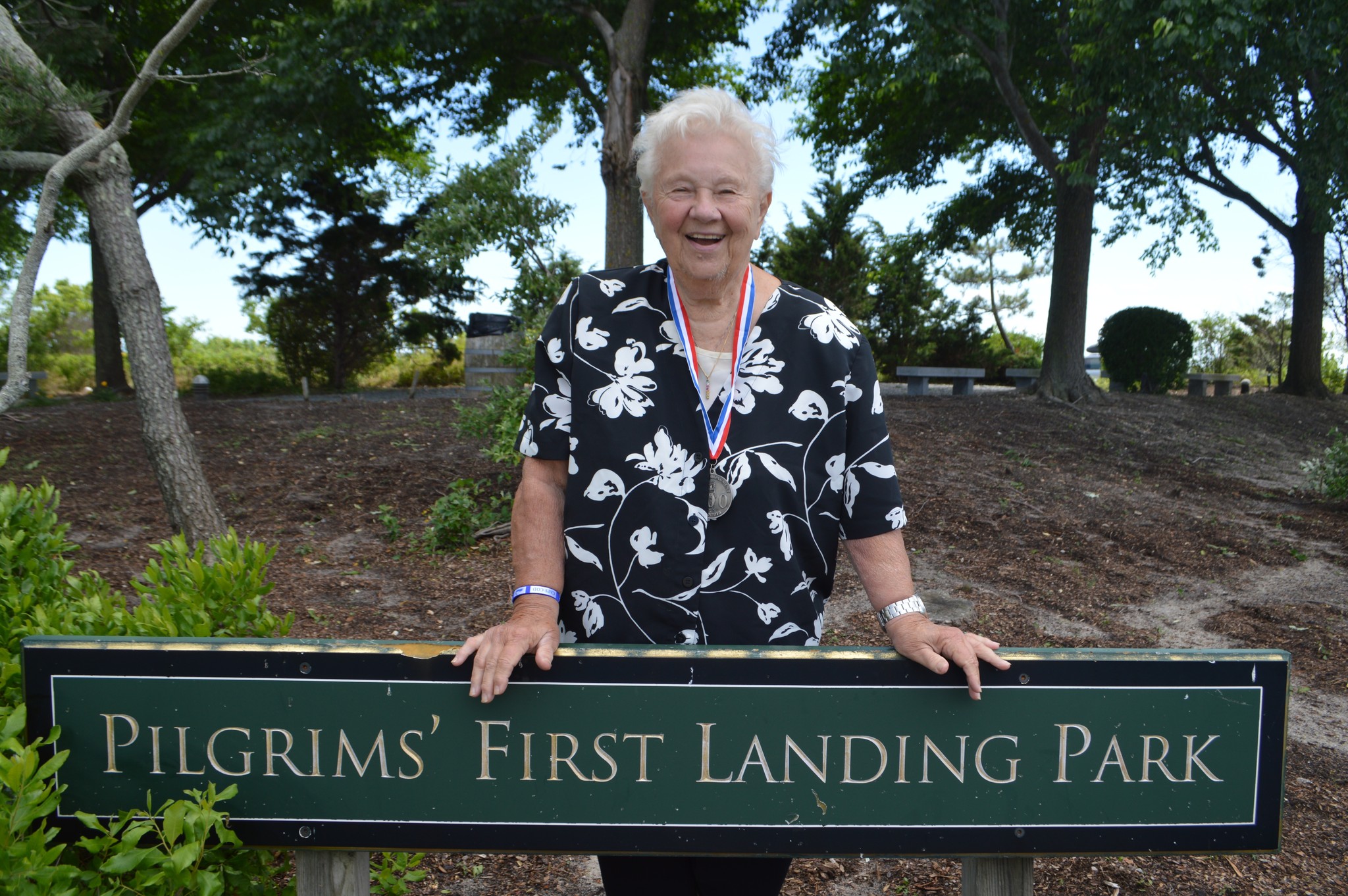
[512, 586, 561, 603]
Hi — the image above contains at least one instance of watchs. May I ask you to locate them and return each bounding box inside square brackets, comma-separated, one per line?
[878, 593, 928, 632]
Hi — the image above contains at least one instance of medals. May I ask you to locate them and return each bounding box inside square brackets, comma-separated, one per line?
[707, 468, 733, 519]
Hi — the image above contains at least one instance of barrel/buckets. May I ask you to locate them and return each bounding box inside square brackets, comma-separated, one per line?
[464, 313, 527, 390]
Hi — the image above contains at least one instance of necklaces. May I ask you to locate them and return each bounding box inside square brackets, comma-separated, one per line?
[451, 85, 1013, 896]
[692, 309, 738, 400]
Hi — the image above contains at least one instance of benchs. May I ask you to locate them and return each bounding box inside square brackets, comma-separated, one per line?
[1005, 368, 1040, 391]
[896, 367, 985, 398]
[1185, 373, 1241, 397]
